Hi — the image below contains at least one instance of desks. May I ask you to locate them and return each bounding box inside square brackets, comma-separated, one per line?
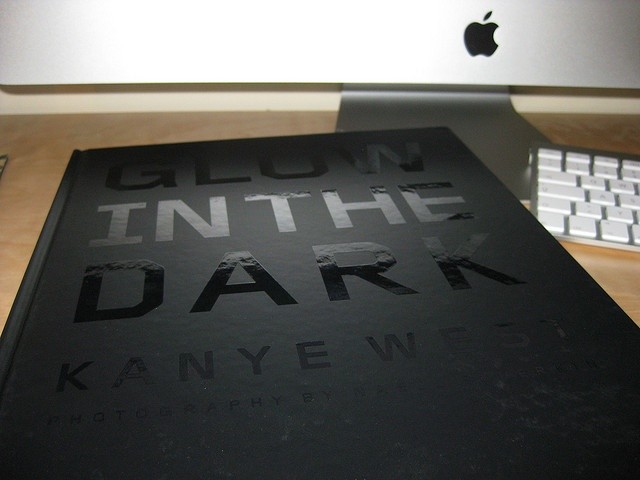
[1, 116, 639, 330]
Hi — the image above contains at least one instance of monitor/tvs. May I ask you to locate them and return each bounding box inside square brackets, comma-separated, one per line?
[1, 0, 640, 203]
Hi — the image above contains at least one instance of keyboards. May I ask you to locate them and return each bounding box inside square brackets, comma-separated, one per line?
[530, 146, 640, 253]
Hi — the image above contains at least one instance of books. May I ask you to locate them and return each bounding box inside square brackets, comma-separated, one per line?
[1, 126, 640, 480]
